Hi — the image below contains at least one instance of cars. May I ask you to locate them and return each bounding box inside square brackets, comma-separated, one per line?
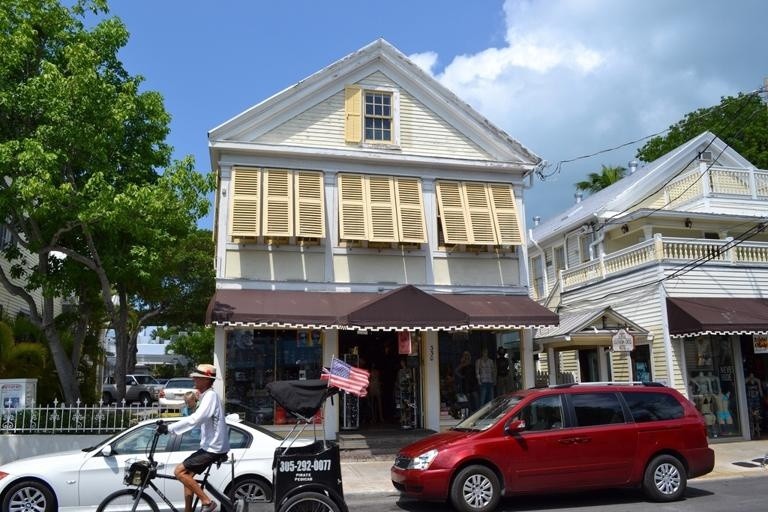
[155, 379, 168, 384]
[0, 415, 325, 511]
[159, 378, 202, 412]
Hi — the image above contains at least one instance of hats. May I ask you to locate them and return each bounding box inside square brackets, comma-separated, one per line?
[188, 363, 222, 381]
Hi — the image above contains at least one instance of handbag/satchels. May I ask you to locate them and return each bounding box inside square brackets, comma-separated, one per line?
[125, 460, 151, 487]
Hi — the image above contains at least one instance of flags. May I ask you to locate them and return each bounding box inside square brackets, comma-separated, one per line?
[326, 356, 370, 398]
[321, 367, 331, 377]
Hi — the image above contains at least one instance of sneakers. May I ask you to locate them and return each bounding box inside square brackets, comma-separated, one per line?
[200, 500, 218, 512]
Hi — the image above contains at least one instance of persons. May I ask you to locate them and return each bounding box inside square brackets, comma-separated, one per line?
[475, 345, 497, 406]
[158, 363, 231, 511]
[691, 371, 764, 432]
[455, 350, 478, 412]
[495, 346, 510, 394]
[179, 392, 202, 436]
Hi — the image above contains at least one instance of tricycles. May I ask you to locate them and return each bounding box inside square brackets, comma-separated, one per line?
[94, 375, 348, 512]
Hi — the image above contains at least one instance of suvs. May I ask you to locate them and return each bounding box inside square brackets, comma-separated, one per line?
[102, 375, 165, 407]
[392, 380, 714, 511]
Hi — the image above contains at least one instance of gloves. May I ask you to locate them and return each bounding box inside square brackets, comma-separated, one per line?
[157, 425, 169, 435]
